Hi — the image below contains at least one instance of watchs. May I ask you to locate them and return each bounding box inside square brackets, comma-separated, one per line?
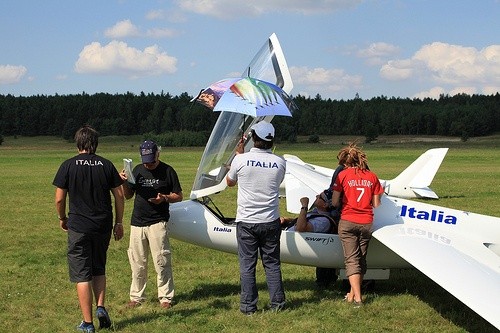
[165, 195, 168, 201]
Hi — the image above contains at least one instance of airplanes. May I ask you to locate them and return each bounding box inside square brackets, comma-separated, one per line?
[166, 34, 500, 333]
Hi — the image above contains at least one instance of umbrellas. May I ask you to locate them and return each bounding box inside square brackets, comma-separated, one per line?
[197, 67, 299, 143]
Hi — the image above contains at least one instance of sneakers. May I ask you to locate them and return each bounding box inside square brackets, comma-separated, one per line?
[77, 321, 95, 333]
[160, 302, 173, 309]
[127, 301, 142, 308]
[97, 307, 111, 330]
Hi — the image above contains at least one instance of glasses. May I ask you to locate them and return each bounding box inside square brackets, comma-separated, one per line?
[319, 194, 326, 203]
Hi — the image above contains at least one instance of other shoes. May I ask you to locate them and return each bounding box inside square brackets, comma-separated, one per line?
[343, 293, 363, 308]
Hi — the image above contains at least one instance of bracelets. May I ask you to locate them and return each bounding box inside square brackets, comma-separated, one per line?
[58, 216, 67, 220]
[301, 207, 308, 210]
[115, 222, 122, 225]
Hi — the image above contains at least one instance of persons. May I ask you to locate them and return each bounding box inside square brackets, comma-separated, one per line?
[281, 190, 339, 233]
[226, 121, 286, 315]
[316, 147, 383, 304]
[119, 141, 183, 308]
[52, 127, 124, 333]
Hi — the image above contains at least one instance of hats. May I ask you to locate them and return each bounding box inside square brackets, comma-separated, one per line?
[251, 120, 275, 141]
[140, 140, 160, 164]
